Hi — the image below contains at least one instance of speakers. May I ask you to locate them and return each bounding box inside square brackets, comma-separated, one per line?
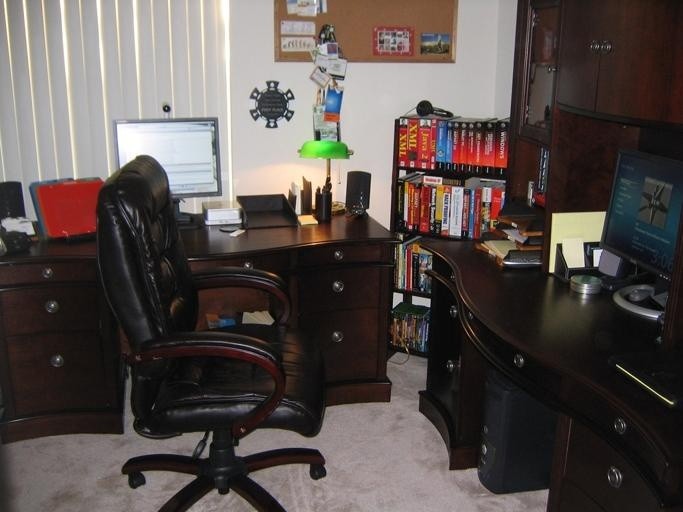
[344, 170, 372, 216]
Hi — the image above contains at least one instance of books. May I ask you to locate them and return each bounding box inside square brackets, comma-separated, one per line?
[394, 244, 433, 292]
[398, 173, 506, 241]
[390, 301, 430, 353]
[202, 201, 243, 227]
[399, 113, 510, 180]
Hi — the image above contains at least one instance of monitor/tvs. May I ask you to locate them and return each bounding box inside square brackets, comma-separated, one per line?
[598, 148, 682, 323]
[113, 116, 223, 222]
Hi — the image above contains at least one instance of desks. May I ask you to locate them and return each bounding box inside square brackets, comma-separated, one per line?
[0, 207, 404, 447]
[419, 237, 682, 511]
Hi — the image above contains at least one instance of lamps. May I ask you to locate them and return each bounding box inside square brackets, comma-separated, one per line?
[298, 141, 355, 216]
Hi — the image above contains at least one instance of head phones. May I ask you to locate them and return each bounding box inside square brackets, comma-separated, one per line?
[416, 100, 453, 117]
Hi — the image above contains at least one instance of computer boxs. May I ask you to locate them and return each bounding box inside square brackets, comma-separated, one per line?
[477, 371, 555, 496]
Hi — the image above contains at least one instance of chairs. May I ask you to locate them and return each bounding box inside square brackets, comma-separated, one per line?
[94, 155, 327, 512]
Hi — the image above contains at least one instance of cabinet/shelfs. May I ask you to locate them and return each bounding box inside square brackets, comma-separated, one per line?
[557, 1, 682, 132]
[389, 115, 512, 358]
[510, 0, 643, 275]
